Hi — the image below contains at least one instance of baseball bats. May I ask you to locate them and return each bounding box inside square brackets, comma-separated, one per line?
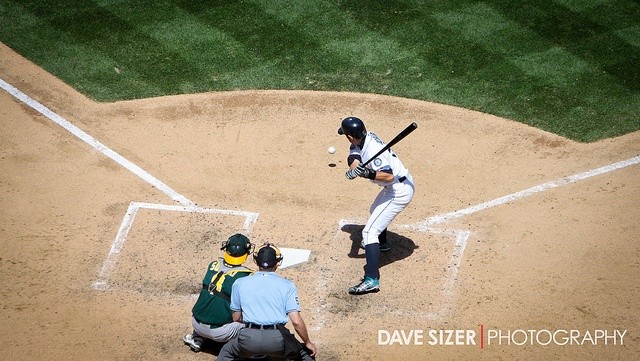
[361, 122, 418, 168]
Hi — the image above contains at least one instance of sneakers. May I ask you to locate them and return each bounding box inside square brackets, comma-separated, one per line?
[183, 334, 203, 354]
[348, 275, 380, 295]
[361, 240, 391, 252]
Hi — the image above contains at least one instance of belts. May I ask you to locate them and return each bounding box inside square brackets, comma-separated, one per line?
[398, 176, 407, 183]
[244, 323, 278, 331]
[193, 316, 223, 330]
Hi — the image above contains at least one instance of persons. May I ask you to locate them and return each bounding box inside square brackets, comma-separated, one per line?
[342, 117, 416, 295]
[217, 243, 316, 361]
[183, 234, 256, 351]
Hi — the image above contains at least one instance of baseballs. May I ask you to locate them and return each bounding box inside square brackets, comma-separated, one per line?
[328, 147, 336, 154]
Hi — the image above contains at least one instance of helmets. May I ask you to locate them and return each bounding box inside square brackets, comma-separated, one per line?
[337, 117, 365, 136]
[253, 242, 283, 269]
[221, 233, 256, 266]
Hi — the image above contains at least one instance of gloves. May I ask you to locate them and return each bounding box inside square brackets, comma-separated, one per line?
[345, 169, 357, 181]
[354, 163, 375, 181]
